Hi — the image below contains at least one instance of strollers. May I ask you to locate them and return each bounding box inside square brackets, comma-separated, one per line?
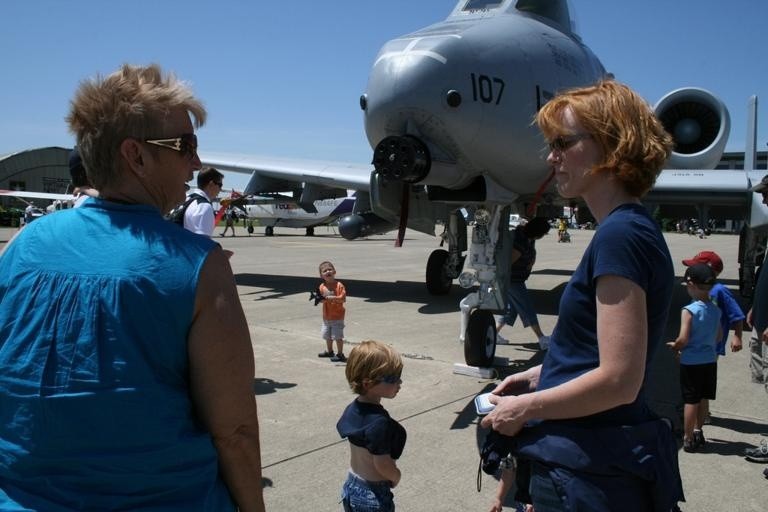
[558, 229, 571, 244]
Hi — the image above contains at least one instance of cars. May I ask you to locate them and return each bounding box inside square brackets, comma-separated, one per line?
[0, 211, 19, 228]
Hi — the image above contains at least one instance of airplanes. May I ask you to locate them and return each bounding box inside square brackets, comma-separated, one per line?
[207, 191, 357, 237]
[0, 189, 78, 216]
[187, 0, 768, 375]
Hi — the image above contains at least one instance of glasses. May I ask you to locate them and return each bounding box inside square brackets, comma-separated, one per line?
[143, 132, 201, 155]
[545, 137, 591, 148]
[213, 180, 223, 186]
[380, 372, 401, 382]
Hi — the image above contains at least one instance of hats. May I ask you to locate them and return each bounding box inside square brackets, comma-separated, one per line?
[685, 261, 717, 285]
[682, 249, 723, 272]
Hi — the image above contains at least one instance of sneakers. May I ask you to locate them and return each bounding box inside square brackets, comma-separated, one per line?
[686, 429, 707, 449]
[330, 354, 346, 362]
[318, 351, 335, 358]
[744, 442, 768, 462]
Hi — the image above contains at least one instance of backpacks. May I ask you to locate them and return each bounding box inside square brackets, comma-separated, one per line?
[165, 196, 209, 225]
